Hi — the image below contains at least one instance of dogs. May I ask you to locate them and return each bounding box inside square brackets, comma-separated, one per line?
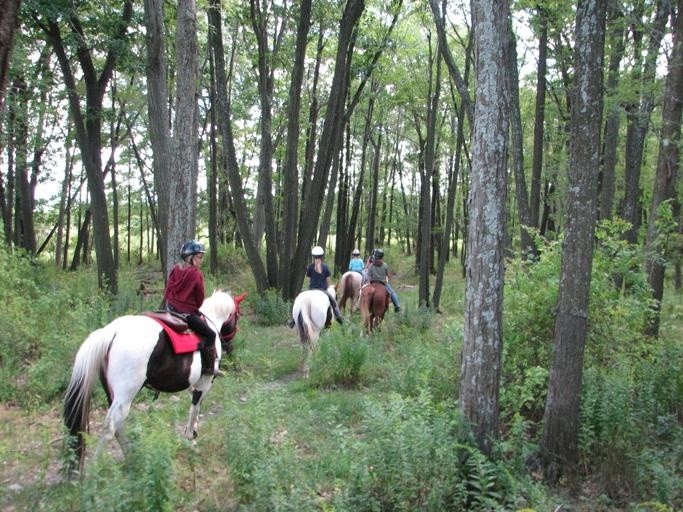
[60, 288, 248, 484]
[336, 270, 390, 337]
[291, 282, 339, 379]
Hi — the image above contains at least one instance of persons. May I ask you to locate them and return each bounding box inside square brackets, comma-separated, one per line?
[348, 249, 365, 274]
[368, 248, 400, 312]
[287, 246, 342, 329]
[163, 240, 224, 377]
[364, 249, 376, 271]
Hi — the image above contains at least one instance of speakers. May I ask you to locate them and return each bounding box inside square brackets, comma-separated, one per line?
[201, 344, 225, 378]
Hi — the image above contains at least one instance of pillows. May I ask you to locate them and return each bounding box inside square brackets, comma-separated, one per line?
[180, 239, 206, 257]
[311, 246, 324, 257]
[373, 249, 384, 258]
[352, 248, 360, 256]
